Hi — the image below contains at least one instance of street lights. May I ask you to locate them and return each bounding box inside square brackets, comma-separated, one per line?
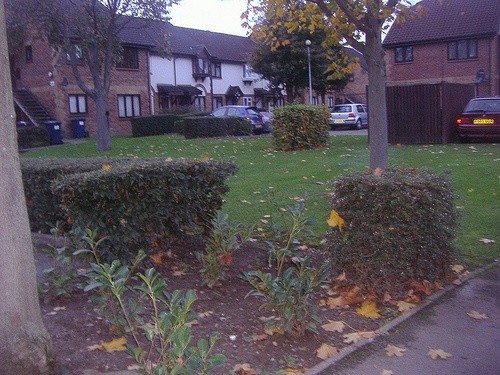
[305, 39, 313, 105]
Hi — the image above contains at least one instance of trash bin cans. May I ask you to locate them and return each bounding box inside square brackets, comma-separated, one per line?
[44, 120, 63, 145]
[17, 121, 29, 128]
[71, 119, 87, 138]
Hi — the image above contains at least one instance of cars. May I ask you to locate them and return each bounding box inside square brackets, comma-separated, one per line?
[205, 106, 274, 135]
[329, 103, 368, 130]
[456, 95, 500, 142]
